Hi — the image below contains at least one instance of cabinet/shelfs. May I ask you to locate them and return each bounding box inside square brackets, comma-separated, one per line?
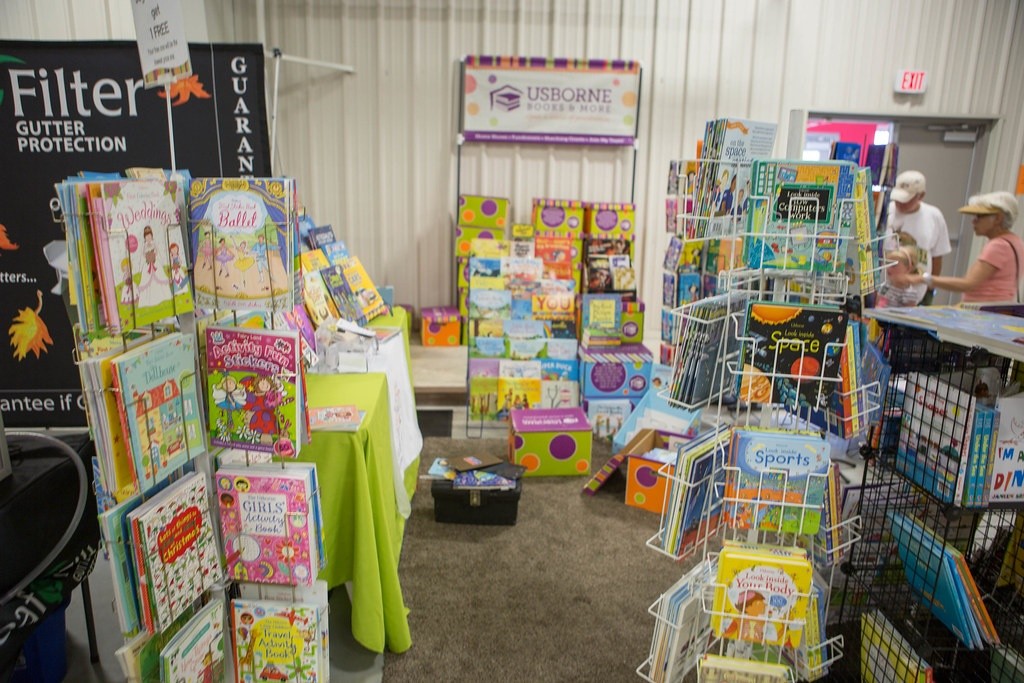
[407, 333, 469, 439]
[57, 170, 330, 683]
[636, 116, 898, 683]
[832, 300, 1024, 683]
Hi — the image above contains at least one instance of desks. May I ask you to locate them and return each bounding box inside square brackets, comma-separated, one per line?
[207, 372, 414, 653]
[332, 304, 425, 507]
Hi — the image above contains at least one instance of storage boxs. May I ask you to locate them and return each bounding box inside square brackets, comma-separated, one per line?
[419, 307, 460, 347]
[508, 409, 593, 477]
[431, 476, 523, 526]
[394, 305, 414, 338]
[583, 428, 697, 516]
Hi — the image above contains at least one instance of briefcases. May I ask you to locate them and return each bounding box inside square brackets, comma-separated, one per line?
[431, 474, 522, 526]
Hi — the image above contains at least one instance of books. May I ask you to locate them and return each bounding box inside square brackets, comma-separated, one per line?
[419, 196, 651, 487]
[51, 167, 402, 683]
[645, 117, 1024, 682]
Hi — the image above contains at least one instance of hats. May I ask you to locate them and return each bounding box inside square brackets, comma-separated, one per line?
[890, 170, 926, 203]
[958, 204, 1004, 214]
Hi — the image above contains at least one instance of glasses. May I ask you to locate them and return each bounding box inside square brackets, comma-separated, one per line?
[976, 214, 990, 221]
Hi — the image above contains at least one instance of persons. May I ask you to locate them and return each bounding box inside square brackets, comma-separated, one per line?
[881, 171, 1023, 310]
